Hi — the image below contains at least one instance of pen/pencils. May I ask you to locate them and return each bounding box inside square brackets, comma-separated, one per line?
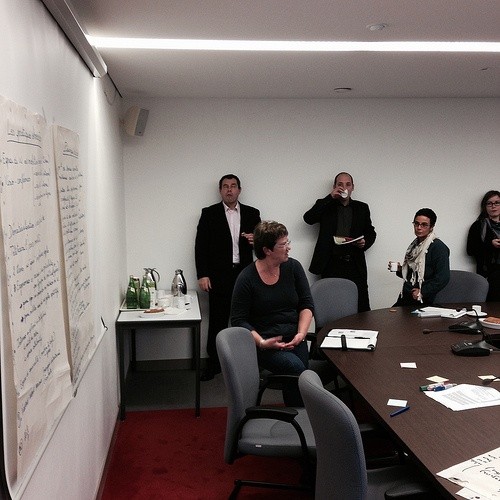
[390, 406, 410, 417]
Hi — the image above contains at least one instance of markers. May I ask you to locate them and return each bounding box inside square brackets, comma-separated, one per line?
[419, 382, 457, 392]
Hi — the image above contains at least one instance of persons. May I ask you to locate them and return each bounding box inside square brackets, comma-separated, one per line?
[302, 171, 378, 315]
[194, 174, 262, 380]
[231, 222, 316, 406]
[467, 190, 500, 303]
[393, 208, 450, 309]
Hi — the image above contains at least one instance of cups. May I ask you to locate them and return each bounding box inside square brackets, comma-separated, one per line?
[391, 263, 398, 271]
[472, 305, 481, 315]
[341, 189, 349, 198]
[150, 287, 193, 308]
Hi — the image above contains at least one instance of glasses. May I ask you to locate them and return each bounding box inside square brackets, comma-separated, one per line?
[274, 240, 291, 248]
[486, 202, 500, 207]
[412, 222, 430, 228]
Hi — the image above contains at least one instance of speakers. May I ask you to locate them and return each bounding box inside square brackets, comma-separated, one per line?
[124, 105, 148, 137]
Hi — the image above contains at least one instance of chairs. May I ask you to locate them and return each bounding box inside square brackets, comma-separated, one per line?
[299, 369, 440, 500]
[312, 277, 359, 384]
[217, 326, 317, 483]
[432, 270, 490, 303]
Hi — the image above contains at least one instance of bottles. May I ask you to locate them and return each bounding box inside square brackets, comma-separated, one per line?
[138, 276, 151, 309]
[126, 275, 138, 309]
[132, 276, 141, 298]
[171, 270, 188, 296]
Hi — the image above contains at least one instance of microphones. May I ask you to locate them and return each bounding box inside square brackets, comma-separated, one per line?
[448, 307, 483, 334]
[466, 307, 500, 348]
[422, 326, 490, 355]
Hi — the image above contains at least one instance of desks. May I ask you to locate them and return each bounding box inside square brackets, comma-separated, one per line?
[116, 290, 202, 416]
[316, 304, 500, 499]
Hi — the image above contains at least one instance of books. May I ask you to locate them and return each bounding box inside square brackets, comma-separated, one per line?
[320, 328, 380, 351]
[334, 235, 364, 244]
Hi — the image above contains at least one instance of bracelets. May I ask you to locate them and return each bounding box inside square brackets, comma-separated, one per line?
[299, 331, 306, 342]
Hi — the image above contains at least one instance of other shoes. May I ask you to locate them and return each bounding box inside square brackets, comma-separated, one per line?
[200, 366, 220, 381]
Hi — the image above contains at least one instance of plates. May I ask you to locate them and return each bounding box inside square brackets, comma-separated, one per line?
[478, 318, 500, 329]
[466, 311, 487, 316]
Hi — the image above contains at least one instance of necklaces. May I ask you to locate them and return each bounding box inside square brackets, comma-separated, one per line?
[262, 261, 277, 277]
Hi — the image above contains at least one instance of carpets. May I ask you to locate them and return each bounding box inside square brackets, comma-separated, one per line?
[98, 404, 316, 500]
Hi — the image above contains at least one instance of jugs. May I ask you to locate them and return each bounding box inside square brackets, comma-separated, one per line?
[143, 268, 161, 289]
